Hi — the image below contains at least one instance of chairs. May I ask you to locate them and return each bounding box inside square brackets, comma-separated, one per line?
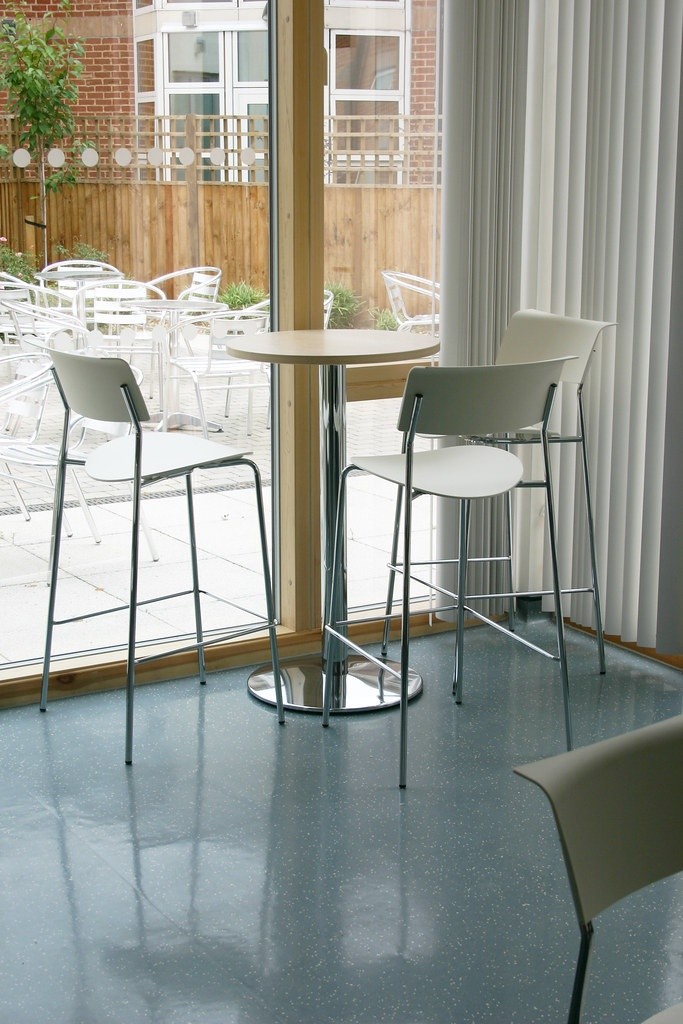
[225, 288, 335, 431]
[1, 271, 78, 356]
[131, 265, 224, 399]
[373, 305, 622, 693]
[33, 259, 124, 351]
[1, 297, 115, 429]
[0, 352, 75, 535]
[379, 270, 442, 359]
[72, 279, 167, 399]
[31, 344, 284, 767]
[0, 361, 158, 563]
[499, 705, 683, 1024]
[157, 311, 276, 436]
[320, 353, 583, 792]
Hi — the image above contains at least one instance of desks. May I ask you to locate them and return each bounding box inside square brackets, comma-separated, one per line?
[35, 269, 125, 353]
[120, 298, 230, 436]
[222, 326, 444, 716]
